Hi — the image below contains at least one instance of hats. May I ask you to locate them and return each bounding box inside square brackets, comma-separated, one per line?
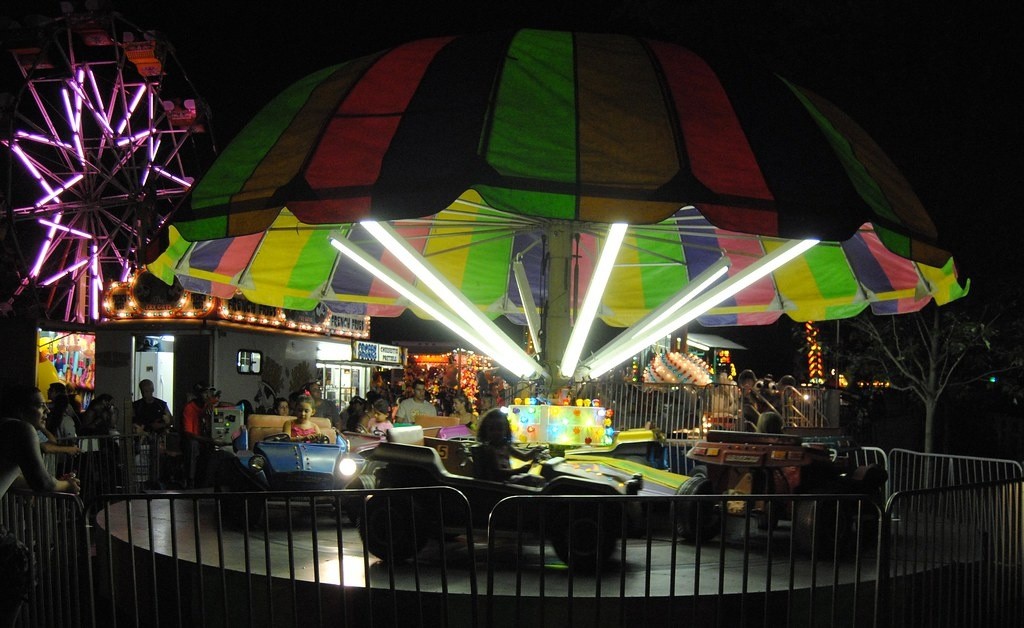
[193, 382, 216, 394]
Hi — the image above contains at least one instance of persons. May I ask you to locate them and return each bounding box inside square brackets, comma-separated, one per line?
[738, 369, 796, 434]
[273, 373, 496, 442]
[182, 382, 220, 436]
[479, 409, 544, 479]
[0, 383, 119, 628]
[131, 380, 173, 484]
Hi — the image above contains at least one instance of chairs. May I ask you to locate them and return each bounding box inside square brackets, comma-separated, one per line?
[706, 429, 802, 449]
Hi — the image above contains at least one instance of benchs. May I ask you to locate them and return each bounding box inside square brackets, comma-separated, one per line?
[414, 415, 459, 439]
[386, 425, 424, 446]
[247, 425, 337, 450]
[436, 425, 471, 441]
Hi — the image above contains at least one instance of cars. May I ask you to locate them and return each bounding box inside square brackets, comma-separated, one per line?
[342, 414, 472, 453]
[685, 428, 892, 563]
[353, 424, 714, 569]
[217, 413, 379, 532]
[560, 419, 670, 471]
